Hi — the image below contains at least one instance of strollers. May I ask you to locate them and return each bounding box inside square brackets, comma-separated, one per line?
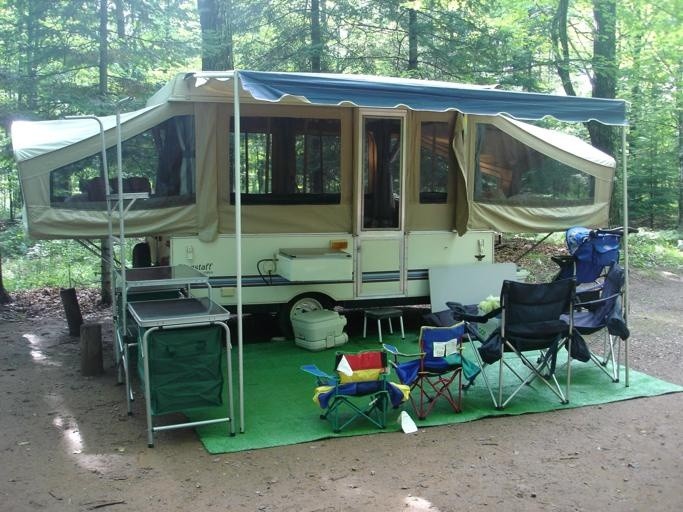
[545, 222, 639, 303]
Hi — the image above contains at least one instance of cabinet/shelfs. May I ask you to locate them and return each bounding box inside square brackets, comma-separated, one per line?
[112, 265, 236, 446]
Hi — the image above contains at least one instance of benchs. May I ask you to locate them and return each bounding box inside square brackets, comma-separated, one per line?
[64, 176, 151, 202]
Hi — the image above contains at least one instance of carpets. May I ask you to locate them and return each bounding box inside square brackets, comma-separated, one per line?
[183, 315, 682, 453]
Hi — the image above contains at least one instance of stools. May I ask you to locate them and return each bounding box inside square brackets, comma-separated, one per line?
[363, 307, 405, 343]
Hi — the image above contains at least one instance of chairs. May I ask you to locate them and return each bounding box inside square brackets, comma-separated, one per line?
[538, 258, 630, 383]
[381, 320, 468, 422]
[298, 349, 413, 434]
[444, 275, 580, 409]
[291, 309, 350, 352]
